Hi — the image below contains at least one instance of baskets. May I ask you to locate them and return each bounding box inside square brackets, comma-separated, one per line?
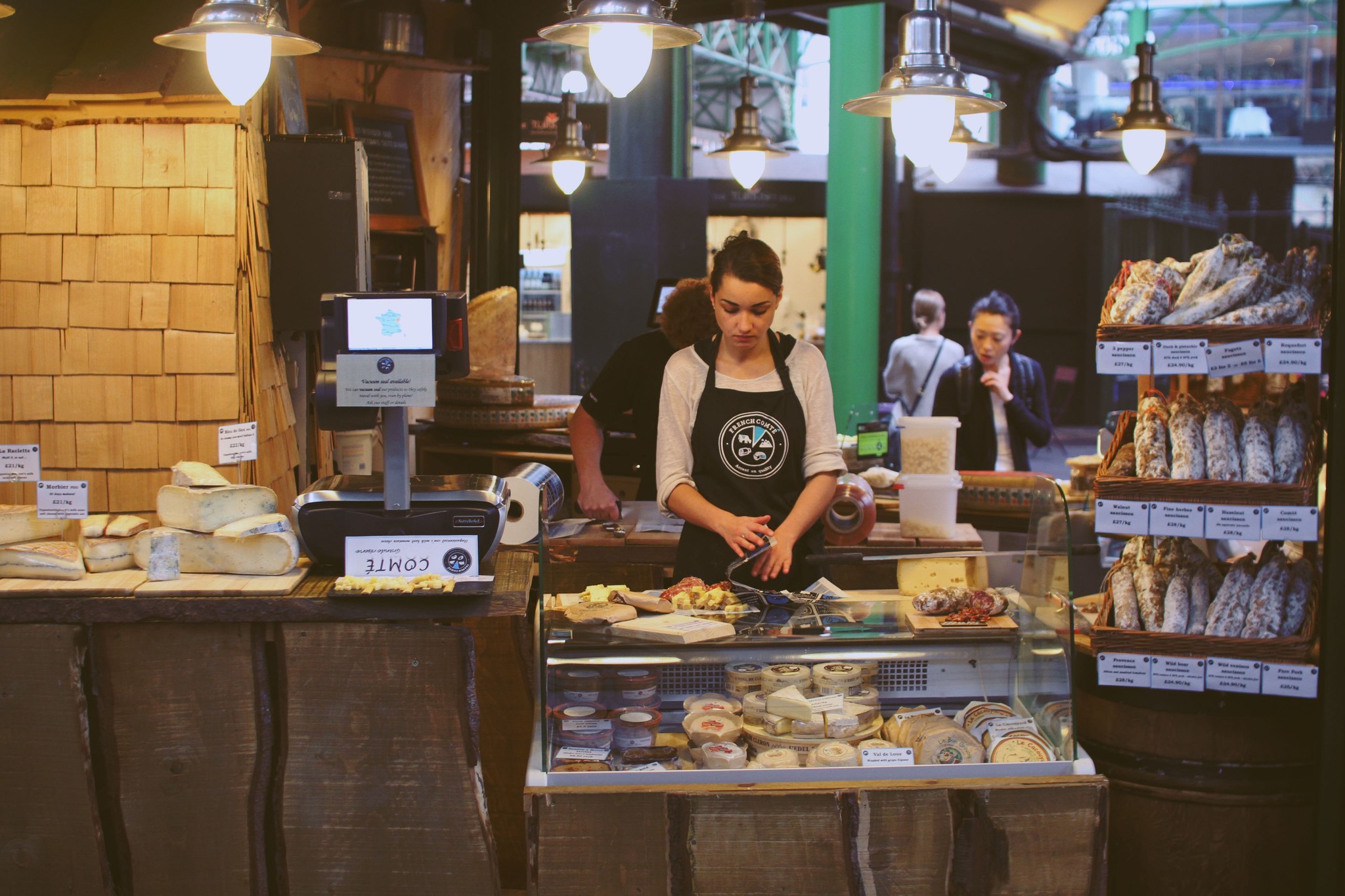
[1096, 258, 1333, 340]
[1093, 410, 1322, 505]
[1090, 586, 1316, 664]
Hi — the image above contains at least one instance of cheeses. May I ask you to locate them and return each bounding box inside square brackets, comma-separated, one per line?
[0, 460, 300, 582]
[539, 647, 1061, 772]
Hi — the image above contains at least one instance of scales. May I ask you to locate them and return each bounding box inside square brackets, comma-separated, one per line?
[293, 294, 507, 577]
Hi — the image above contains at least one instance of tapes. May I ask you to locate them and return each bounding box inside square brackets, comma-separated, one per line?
[490, 462, 596, 545]
[820, 472, 877, 546]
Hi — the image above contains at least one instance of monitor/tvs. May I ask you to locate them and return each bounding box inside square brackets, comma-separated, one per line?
[336, 292, 447, 353]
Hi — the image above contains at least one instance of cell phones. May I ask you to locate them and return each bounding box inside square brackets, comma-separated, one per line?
[574, 500, 623, 516]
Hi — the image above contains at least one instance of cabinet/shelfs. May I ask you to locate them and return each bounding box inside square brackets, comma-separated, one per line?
[1091, 257, 1332, 661]
[526, 467, 1091, 785]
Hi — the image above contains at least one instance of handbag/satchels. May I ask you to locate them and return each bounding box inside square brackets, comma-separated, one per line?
[883, 428, 903, 470]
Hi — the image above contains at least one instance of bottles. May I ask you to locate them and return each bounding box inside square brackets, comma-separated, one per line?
[521, 270, 561, 311]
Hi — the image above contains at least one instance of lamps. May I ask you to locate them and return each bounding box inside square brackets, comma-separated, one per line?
[152, 0, 322, 107]
[928, 116, 992, 184]
[1090, 0, 1194, 175]
[531, 44, 603, 195]
[708, 2, 789, 189]
[536, 0, 701, 97]
[844, 0, 1005, 168]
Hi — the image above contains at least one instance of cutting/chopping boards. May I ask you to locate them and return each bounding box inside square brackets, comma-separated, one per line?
[905, 612, 1019, 636]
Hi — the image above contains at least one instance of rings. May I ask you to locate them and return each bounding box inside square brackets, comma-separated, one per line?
[741, 529, 751, 538]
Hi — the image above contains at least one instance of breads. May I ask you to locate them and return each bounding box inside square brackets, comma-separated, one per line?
[1123, 231, 1323, 334]
[1132, 378, 1306, 483]
[1101, 532, 1312, 643]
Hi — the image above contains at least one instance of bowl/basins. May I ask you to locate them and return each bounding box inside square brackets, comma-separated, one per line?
[682, 692, 748, 770]
[894, 416, 964, 536]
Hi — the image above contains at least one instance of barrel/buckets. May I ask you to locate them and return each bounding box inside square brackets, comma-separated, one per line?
[336, 429, 379, 475]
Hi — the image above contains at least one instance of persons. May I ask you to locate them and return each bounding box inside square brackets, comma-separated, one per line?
[567, 272, 718, 521]
[656, 231, 846, 598]
[932, 290, 1053, 472]
[883, 285, 965, 471]
